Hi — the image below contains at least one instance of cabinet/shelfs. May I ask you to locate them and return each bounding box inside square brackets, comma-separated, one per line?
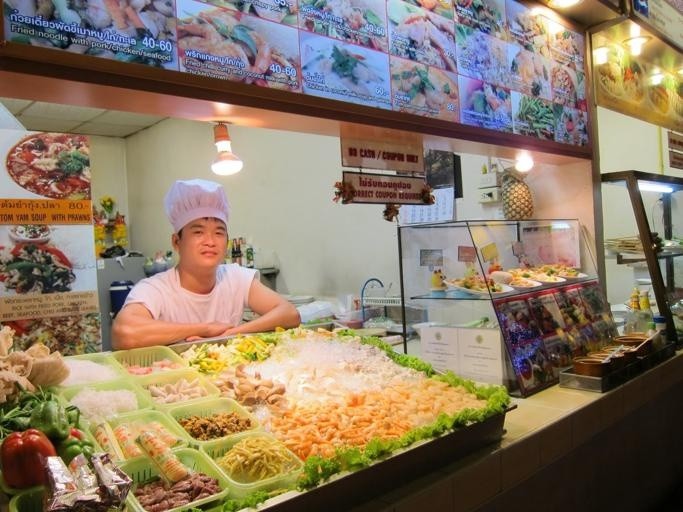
[398, 169, 682, 398]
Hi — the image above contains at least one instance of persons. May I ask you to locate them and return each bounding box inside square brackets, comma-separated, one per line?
[105, 177, 300, 351]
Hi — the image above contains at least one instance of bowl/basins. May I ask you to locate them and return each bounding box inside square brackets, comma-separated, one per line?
[411, 321, 448, 338]
[518, 356, 534, 388]
[573, 334, 650, 375]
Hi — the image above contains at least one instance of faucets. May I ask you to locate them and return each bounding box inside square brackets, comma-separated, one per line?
[360, 278, 384, 321]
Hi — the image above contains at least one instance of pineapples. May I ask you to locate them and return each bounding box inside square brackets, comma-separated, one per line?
[501, 165, 535, 220]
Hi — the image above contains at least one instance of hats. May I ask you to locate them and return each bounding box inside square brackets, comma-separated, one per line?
[161, 178, 230, 234]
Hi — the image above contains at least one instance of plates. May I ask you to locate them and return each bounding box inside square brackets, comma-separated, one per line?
[443, 263, 589, 296]
[8, 224, 53, 245]
[289, 295, 314, 305]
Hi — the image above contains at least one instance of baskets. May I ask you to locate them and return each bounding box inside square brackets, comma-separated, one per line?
[1, 344, 307, 512]
[0, 344, 307, 512]
[362, 296, 402, 307]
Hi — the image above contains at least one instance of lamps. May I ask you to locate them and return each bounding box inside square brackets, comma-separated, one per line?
[210, 121, 243, 177]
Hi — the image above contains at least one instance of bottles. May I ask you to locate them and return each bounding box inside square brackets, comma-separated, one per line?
[225, 237, 253, 268]
[624, 287, 654, 332]
[646, 321, 662, 347]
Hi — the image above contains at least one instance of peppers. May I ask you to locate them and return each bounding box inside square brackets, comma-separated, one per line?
[0, 398, 95, 488]
